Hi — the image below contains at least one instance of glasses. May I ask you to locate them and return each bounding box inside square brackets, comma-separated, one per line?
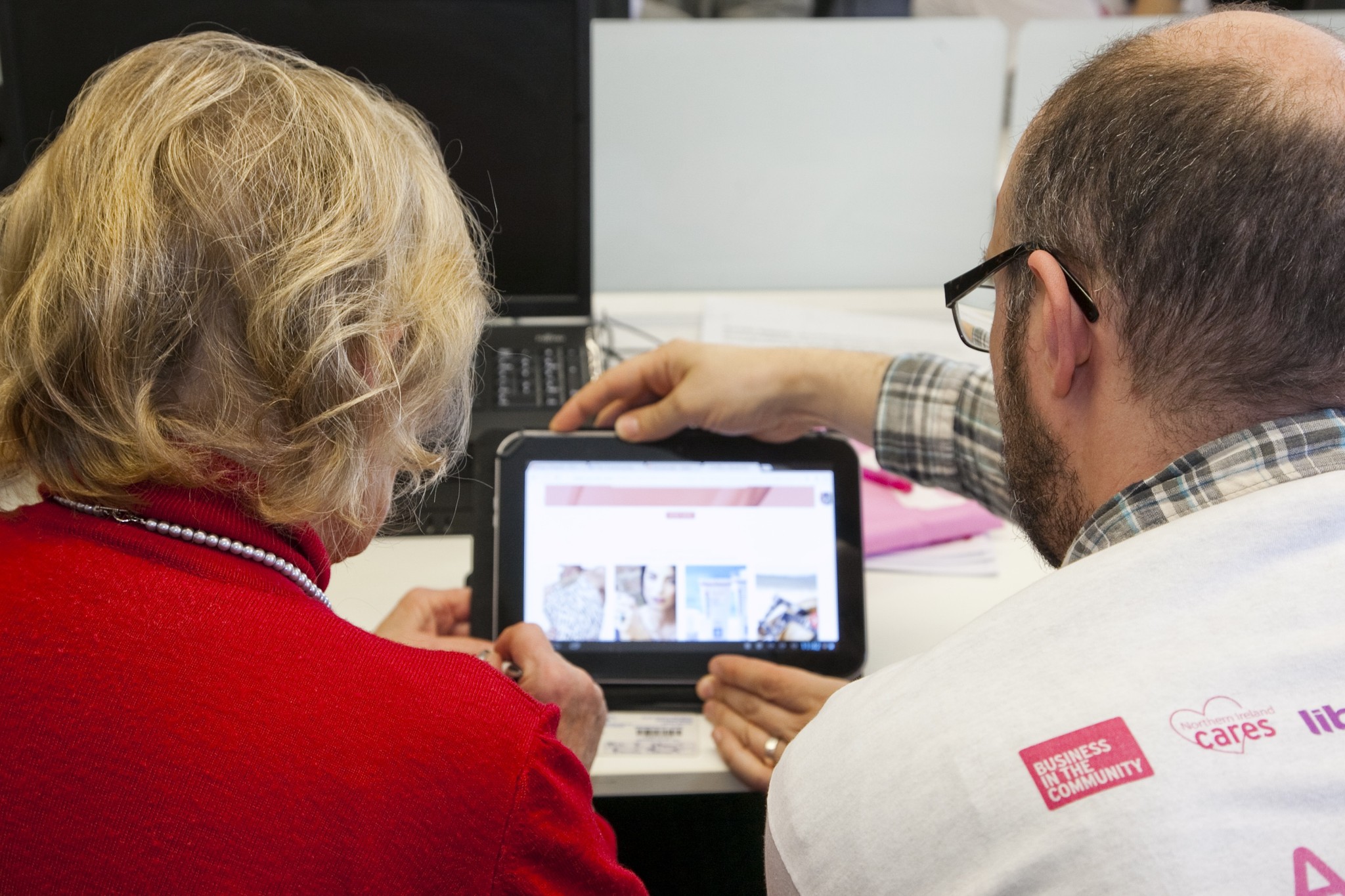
[943, 240, 1101, 353]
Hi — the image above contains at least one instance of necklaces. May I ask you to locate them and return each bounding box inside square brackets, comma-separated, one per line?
[44, 490, 345, 622]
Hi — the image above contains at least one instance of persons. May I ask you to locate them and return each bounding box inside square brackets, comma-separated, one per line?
[548, 4, 1345, 896]
[0, 30, 647, 896]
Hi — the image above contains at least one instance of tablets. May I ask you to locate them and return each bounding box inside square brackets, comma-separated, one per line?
[486, 430, 861, 705]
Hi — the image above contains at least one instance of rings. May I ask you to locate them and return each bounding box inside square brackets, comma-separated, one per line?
[763, 737, 779, 767]
[478, 649, 489, 661]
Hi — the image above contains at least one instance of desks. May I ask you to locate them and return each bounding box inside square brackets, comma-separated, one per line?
[327, 521, 1060, 795]
